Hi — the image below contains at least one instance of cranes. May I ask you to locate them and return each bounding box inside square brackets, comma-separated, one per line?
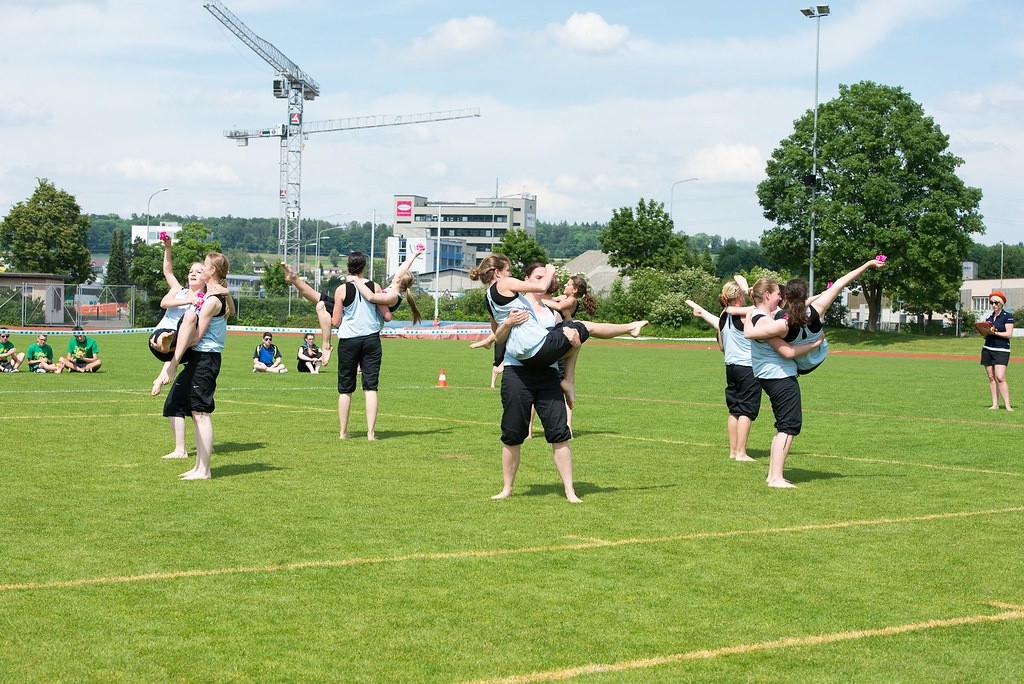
[203, 0, 481, 276]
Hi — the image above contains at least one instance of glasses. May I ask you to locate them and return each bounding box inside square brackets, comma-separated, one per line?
[264, 338, 272, 341]
[989, 301, 999, 305]
[0, 335, 9, 338]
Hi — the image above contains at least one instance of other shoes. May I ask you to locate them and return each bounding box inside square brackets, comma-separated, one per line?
[11, 368, 18, 372]
[2, 367, 11, 372]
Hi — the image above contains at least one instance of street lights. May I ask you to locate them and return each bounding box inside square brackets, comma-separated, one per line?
[799, 5, 831, 297]
[492, 192, 530, 252]
[317, 226, 346, 292]
[999, 240, 1004, 292]
[147, 189, 168, 245]
[315, 212, 349, 291]
[304, 237, 330, 283]
[295, 243, 317, 270]
[671, 177, 697, 220]
[371, 209, 376, 281]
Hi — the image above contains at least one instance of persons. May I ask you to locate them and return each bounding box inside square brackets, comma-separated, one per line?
[252, 332, 288, 373]
[469, 254, 649, 504]
[683, 259, 886, 489]
[974, 290, 1015, 412]
[58, 326, 102, 373]
[148, 232, 235, 481]
[297, 334, 323, 374]
[0, 327, 26, 373]
[26, 334, 66, 374]
[281, 243, 425, 442]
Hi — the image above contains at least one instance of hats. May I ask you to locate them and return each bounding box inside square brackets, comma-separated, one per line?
[989, 289, 1007, 304]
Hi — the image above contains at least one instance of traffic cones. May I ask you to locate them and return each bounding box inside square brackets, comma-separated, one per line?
[437, 368, 447, 387]
